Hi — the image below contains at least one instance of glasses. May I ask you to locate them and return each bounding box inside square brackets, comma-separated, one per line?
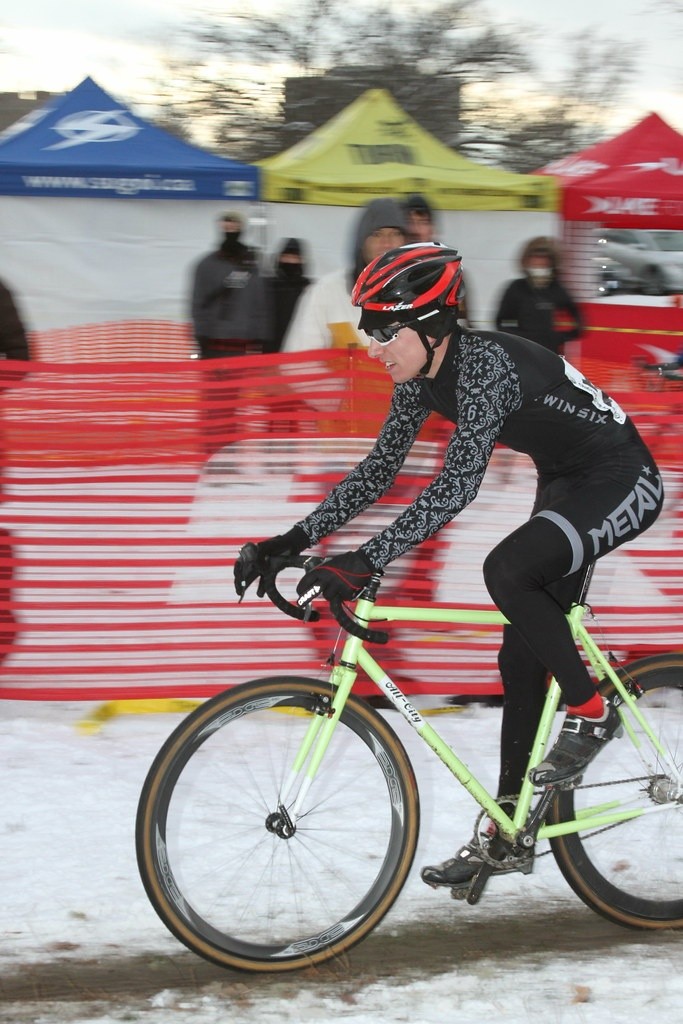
[364, 310, 440, 346]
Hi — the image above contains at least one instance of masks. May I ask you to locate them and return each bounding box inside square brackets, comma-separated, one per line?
[527, 269, 552, 284]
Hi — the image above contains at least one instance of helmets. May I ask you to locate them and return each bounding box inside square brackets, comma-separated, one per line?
[352, 241, 467, 330]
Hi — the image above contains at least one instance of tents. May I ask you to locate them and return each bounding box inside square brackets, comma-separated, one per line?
[248, 87, 560, 212]
[1, 75, 260, 200]
[530, 110, 683, 229]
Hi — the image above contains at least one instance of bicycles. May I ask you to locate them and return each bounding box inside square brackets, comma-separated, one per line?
[131, 555, 683, 976]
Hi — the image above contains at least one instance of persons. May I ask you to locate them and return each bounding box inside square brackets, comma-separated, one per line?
[192, 212, 267, 469]
[0, 279, 31, 396]
[280, 197, 418, 418]
[269, 240, 312, 438]
[235, 240, 666, 888]
[404, 194, 432, 240]
[495, 238, 586, 355]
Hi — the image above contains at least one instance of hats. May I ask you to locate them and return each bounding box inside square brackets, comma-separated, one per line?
[356, 199, 412, 259]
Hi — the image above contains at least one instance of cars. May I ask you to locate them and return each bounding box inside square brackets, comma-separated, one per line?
[594, 228, 683, 295]
[594, 258, 632, 295]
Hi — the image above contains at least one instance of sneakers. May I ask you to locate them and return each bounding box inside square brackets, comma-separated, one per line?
[421, 832, 512, 887]
[527, 696, 621, 787]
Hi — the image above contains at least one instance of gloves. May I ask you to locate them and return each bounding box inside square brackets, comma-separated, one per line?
[234, 525, 314, 598]
[296, 547, 378, 608]
